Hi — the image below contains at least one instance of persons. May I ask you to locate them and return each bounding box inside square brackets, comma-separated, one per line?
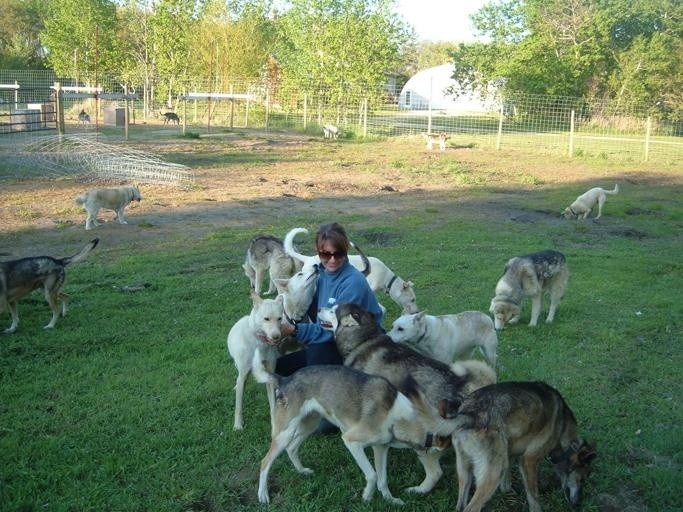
[275, 223, 387, 433]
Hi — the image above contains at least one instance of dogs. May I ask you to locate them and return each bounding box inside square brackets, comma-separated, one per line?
[560, 183, 619, 220]
[242, 233, 292, 295]
[386, 304, 496, 365]
[227, 288, 284, 432]
[76, 109, 91, 129]
[160, 111, 179, 126]
[76, 184, 142, 230]
[488, 249, 569, 331]
[258, 364, 450, 505]
[284, 227, 420, 314]
[323, 124, 340, 139]
[273, 264, 320, 323]
[317, 301, 495, 419]
[406, 375, 598, 512]
[422, 133, 451, 151]
[0, 237, 100, 334]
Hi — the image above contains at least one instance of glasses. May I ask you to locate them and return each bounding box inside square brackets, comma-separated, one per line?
[317, 248, 347, 261]
